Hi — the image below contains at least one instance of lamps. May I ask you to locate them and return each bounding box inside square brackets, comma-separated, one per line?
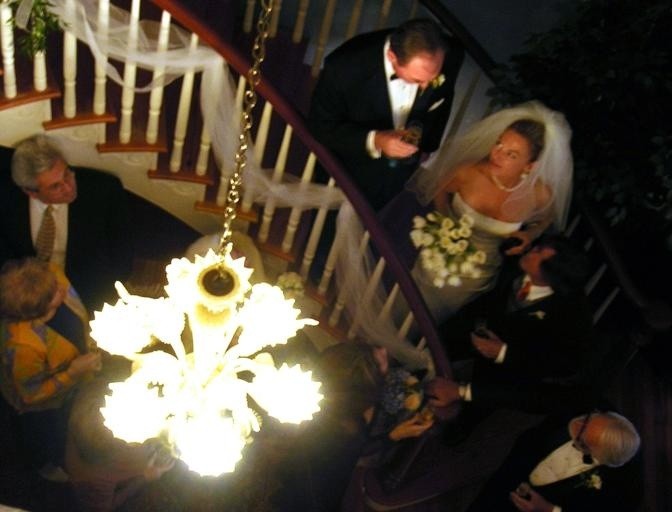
[89, 0, 326, 480]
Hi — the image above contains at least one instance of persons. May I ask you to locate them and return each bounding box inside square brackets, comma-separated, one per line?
[0, 133, 132, 298]
[0, 257, 103, 483]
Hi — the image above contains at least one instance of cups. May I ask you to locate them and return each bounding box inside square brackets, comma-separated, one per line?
[474, 320, 486, 338]
[516, 481, 530, 498]
[420, 403, 436, 423]
[404, 120, 423, 146]
[499, 236, 522, 255]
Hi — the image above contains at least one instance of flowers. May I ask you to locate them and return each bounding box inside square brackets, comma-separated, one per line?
[381, 366, 427, 417]
[273, 268, 306, 303]
[410, 209, 488, 291]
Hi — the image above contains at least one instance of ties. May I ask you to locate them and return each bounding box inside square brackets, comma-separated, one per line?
[34, 204, 56, 262]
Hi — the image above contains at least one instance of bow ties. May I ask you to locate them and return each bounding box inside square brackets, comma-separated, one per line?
[518, 281, 533, 301]
